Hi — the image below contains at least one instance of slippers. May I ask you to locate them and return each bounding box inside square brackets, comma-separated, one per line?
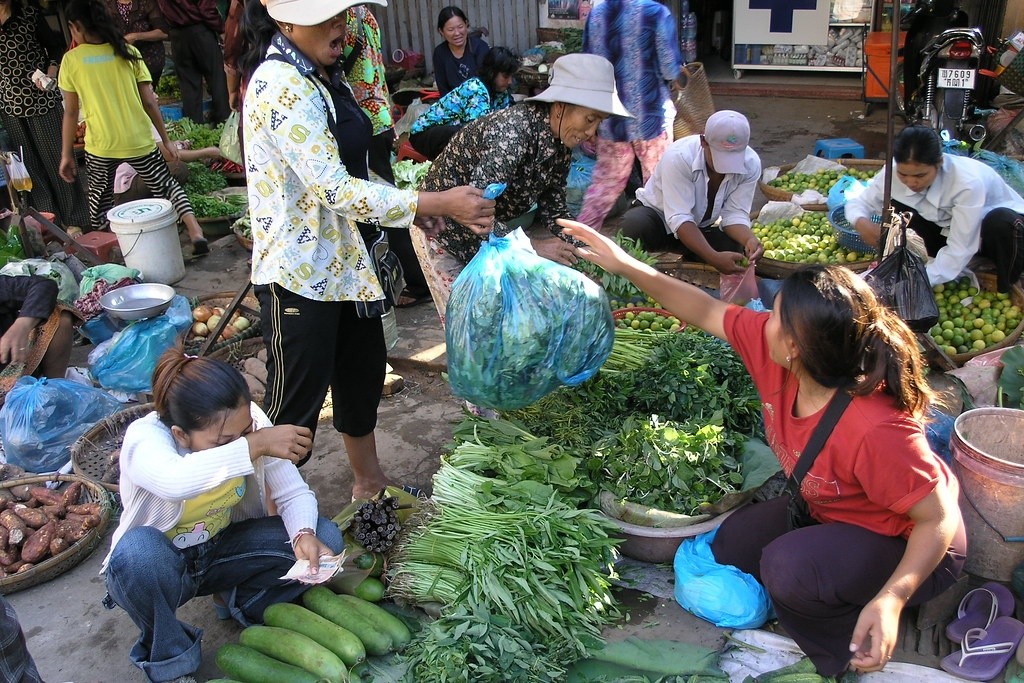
[940, 615, 1024, 682]
[945, 580, 1015, 645]
[191, 238, 209, 257]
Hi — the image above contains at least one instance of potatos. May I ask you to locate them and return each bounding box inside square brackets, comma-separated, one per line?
[241, 348, 267, 402]
[1, 443, 121, 580]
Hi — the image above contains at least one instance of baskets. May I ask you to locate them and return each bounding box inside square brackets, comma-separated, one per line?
[666, 62, 716, 143]
[827, 203, 884, 256]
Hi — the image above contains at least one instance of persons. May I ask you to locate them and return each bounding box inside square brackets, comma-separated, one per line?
[242, 0, 502, 528]
[618, 108, 767, 274]
[429, 2, 496, 94]
[160, 0, 231, 126]
[402, 46, 521, 160]
[101, 83, 220, 205]
[405, 51, 624, 421]
[100, 0, 166, 87]
[551, 209, 976, 683]
[94, 349, 348, 683]
[1, 0, 93, 231]
[0, 272, 83, 401]
[567, 1, 696, 234]
[58, 0, 212, 257]
[840, 123, 1024, 292]
[332, 2, 430, 308]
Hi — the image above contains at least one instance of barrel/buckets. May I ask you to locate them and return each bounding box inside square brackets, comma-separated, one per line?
[948, 407, 1024, 582]
[107, 198, 187, 285]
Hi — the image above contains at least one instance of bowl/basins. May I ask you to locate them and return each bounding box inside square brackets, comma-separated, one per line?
[594, 507, 745, 563]
[101, 281, 177, 318]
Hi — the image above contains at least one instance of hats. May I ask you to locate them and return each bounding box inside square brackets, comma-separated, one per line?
[523, 53, 639, 121]
[705, 110, 751, 175]
[265, 0, 388, 26]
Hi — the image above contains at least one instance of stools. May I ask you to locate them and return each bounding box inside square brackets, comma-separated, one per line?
[67, 231, 122, 265]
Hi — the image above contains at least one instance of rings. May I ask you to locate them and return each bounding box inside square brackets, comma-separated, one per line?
[20, 347, 26, 351]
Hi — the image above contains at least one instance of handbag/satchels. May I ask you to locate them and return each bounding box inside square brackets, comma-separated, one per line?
[753, 467, 809, 513]
[5, 154, 33, 192]
[444, 183, 615, 412]
[862, 216, 940, 334]
[0, 375, 124, 474]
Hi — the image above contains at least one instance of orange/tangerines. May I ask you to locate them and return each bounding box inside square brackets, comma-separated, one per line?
[751, 165, 1021, 356]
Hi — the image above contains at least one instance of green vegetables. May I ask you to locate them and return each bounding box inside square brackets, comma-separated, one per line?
[160, 116, 226, 149]
[182, 160, 246, 217]
[542, 26, 583, 54]
[391, 157, 432, 192]
[380, 233, 762, 683]
